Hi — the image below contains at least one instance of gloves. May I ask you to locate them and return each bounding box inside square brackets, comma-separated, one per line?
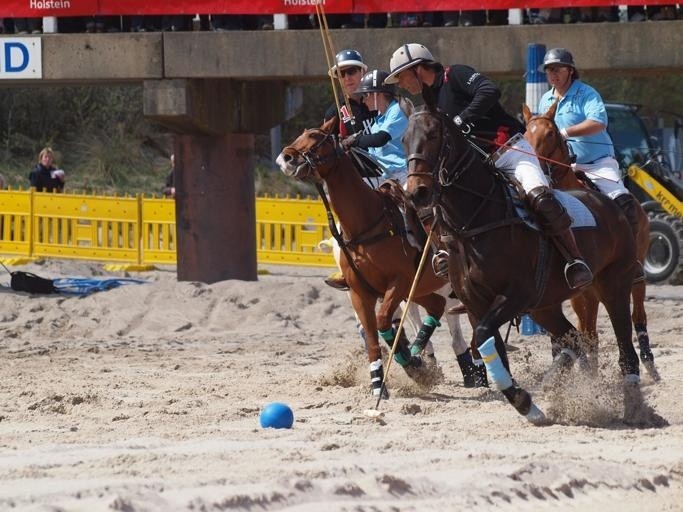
[341, 133, 356, 153]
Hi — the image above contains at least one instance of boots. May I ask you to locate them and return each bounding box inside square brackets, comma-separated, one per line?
[615, 194, 647, 282]
[528, 187, 593, 288]
[326, 274, 348, 288]
[422, 217, 449, 274]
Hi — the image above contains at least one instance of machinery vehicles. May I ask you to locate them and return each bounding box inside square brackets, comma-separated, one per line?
[600, 103, 683, 285]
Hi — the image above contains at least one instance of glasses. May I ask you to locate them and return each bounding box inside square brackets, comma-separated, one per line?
[337, 64, 357, 78]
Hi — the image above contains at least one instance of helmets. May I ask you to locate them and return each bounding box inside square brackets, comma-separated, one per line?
[327, 50, 368, 78]
[537, 48, 576, 72]
[354, 69, 395, 94]
[384, 44, 435, 84]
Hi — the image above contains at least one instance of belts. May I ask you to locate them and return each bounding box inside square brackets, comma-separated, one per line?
[490, 134, 522, 160]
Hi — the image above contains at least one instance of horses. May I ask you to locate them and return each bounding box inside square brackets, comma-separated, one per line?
[398, 81, 641, 426]
[317, 221, 474, 389]
[522, 96, 661, 381]
[275, 115, 450, 400]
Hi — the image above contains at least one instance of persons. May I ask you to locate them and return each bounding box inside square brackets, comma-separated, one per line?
[529, 43, 647, 286]
[381, 42, 596, 292]
[323, 68, 451, 291]
[29, 147, 65, 266]
[163, 151, 176, 250]
[319, 47, 380, 146]
[1, 1, 682, 37]
